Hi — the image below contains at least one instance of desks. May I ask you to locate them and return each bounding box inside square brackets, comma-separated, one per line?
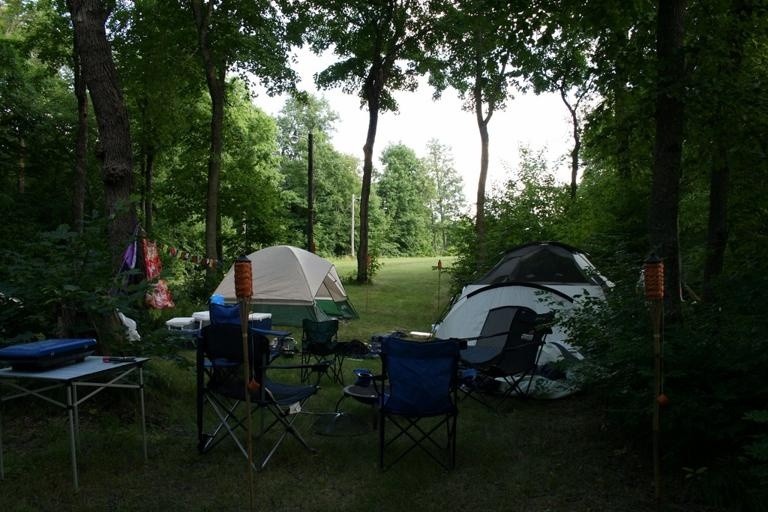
[336, 382, 382, 430]
[0, 354, 162, 490]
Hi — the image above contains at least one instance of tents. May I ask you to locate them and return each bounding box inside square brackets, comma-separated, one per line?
[429, 239, 621, 402]
[209, 244, 360, 326]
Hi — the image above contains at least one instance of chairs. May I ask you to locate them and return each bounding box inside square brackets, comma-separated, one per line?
[301, 319, 351, 387]
[188, 319, 321, 470]
[455, 305, 555, 417]
[376, 334, 469, 475]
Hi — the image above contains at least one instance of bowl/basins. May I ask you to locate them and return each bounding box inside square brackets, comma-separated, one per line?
[343, 383, 387, 405]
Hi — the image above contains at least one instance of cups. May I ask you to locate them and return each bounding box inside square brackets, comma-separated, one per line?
[356, 371, 371, 387]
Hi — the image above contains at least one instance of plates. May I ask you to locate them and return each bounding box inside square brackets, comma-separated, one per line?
[408, 331, 432, 339]
[102, 357, 135, 363]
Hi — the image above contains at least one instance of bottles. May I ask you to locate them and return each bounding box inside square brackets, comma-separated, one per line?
[282, 336, 294, 358]
[430, 323, 435, 333]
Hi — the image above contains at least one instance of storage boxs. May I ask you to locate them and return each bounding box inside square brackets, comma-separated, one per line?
[165, 316, 194, 333]
[248, 312, 270, 331]
[191, 310, 211, 328]
